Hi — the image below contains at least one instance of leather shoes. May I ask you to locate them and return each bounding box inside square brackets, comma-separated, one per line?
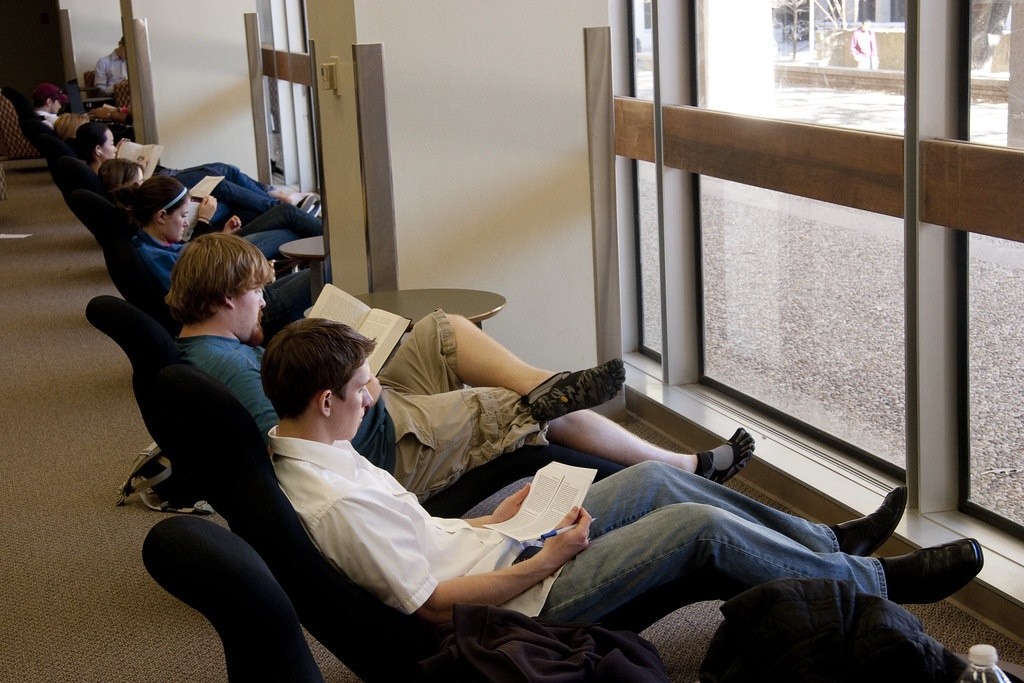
[879, 536, 985, 606]
[836, 485, 907, 558]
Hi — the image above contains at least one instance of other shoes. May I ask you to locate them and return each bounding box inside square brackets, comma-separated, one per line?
[521, 357, 626, 421]
[295, 192, 314, 210]
[304, 199, 322, 218]
[694, 428, 755, 484]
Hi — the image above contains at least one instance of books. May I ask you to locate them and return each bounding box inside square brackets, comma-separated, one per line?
[307, 284, 412, 378]
[115, 140, 163, 181]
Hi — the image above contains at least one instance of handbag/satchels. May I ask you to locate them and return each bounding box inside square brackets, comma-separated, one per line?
[114, 438, 213, 514]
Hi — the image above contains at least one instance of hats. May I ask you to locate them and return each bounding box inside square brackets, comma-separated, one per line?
[31, 83, 68, 103]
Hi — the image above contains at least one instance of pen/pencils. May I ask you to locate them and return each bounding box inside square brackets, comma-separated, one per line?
[541, 518, 599, 540]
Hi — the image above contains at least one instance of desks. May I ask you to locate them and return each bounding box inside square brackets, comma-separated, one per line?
[279, 227, 326, 291]
[82, 95, 110, 109]
[300, 285, 509, 346]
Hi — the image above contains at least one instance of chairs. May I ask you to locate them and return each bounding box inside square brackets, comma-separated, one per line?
[47, 153, 112, 205]
[112, 79, 130, 108]
[85, 288, 628, 517]
[66, 184, 193, 295]
[84, 68, 97, 87]
[126, 354, 750, 680]
[2, 87, 75, 149]
[129, 500, 353, 683]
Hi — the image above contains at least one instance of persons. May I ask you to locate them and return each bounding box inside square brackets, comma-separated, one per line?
[160, 235, 755, 522]
[29, 33, 316, 227]
[259, 318, 986, 631]
[115, 177, 312, 331]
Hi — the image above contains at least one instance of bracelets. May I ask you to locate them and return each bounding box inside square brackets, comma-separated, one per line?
[198, 216, 210, 222]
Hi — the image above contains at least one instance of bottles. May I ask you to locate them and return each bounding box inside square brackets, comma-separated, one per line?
[961, 644, 1013, 683]
